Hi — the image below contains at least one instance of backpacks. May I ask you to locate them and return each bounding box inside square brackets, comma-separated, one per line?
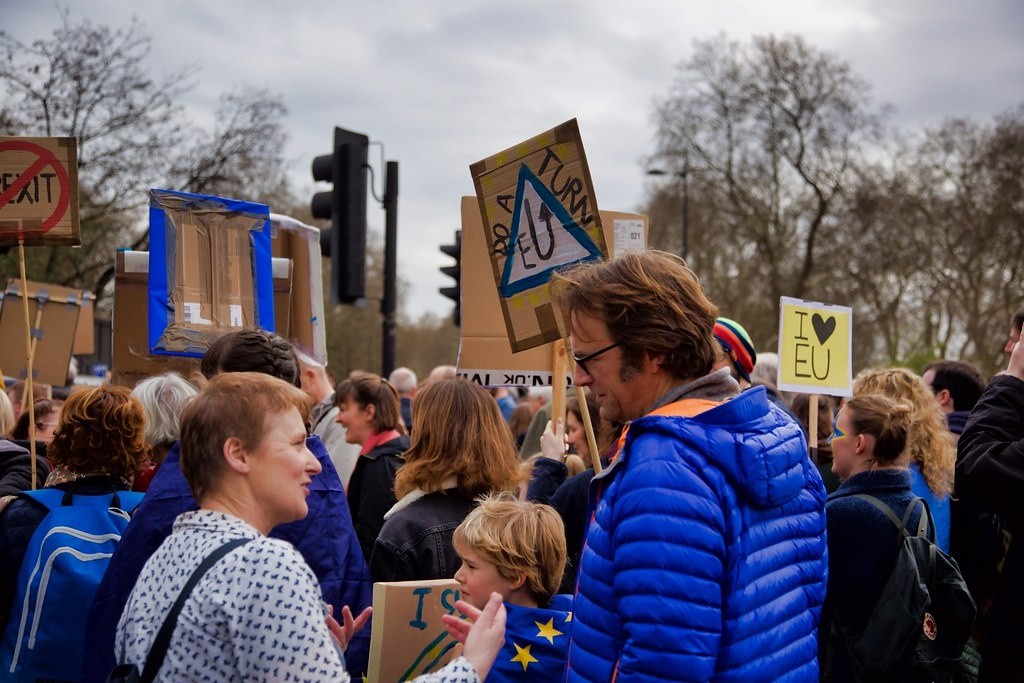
[833, 494, 977, 683]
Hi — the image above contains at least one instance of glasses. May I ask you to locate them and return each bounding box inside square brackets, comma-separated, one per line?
[574, 342, 621, 376]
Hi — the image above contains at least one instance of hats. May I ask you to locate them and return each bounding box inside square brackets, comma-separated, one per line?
[713, 317, 756, 384]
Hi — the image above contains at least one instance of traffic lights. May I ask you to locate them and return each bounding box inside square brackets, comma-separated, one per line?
[310, 126, 369, 304]
[439, 230, 462, 327]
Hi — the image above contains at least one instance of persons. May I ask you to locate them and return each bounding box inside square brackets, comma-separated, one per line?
[0, 310, 1024, 683]
[549, 252, 832, 683]
